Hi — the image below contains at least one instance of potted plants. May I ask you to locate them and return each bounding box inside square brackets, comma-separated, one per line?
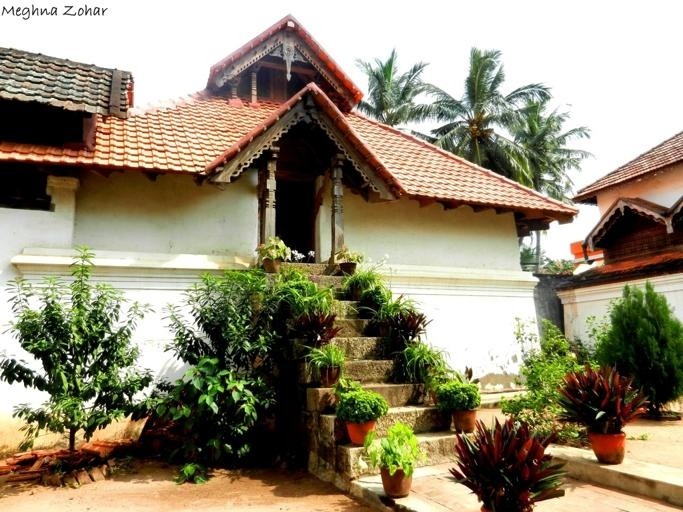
[557, 365, 652, 463]
[254, 237, 296, 275]
[448, 416, 565, 512]
[361, 421, 423, 500]
[332, 242, 367, 277]
[433, 367, 483, 434]
[301, 345, 348, 389]
[345, 261, 444, 380]
[333, 389, 389, 446]
